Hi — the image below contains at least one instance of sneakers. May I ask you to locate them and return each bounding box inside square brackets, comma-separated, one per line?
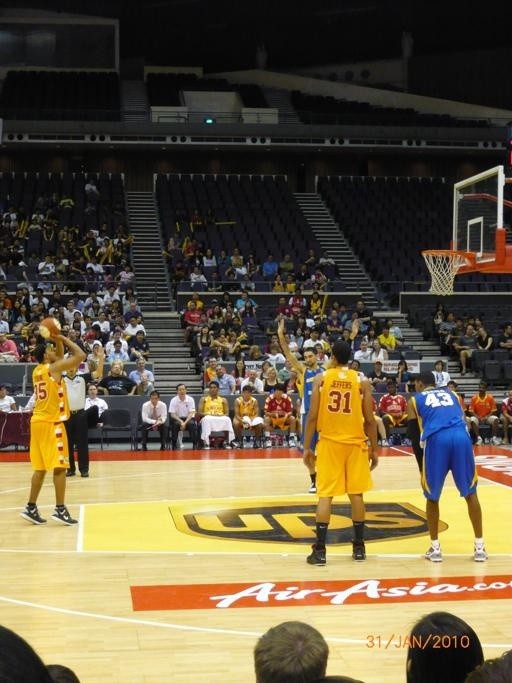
[51, 507, 78, 524]
[474, 542, 488, 560]
[475, 435, 510, 446]
[426, 543, 442, 561]
[201, 437, 296, 449]
[20, 503, 46, 524]
[353, 538, 365, 560]
[65, 471, 75, 476]
[307, 543, 326, 565]
[367, 436, 412, 447]
[309, 478, 316, 492]
[81, 471, 88, 476]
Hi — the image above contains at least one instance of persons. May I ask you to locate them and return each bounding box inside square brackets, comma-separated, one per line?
[434, 303, 511, 375]
[162, 204, 452, 395]
[20, 331, 88, 527]
[301, 339, 382, 566]
[404, 370, 490, 565]
[2, 178, 155, 426]
[141, 382, 512, 450]
[403, 611, 484, 683]
[62, 366, 92, 480]
[275, 318, 334, 494]
[251, 618, 331, 683]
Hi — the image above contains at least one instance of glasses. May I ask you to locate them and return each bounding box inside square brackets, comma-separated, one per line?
[89, 388, 96, 390]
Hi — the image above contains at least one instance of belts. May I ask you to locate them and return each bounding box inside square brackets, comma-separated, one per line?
[69, 408, 84, 414]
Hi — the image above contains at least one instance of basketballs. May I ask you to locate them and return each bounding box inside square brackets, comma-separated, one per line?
[39, 317, 61, 339]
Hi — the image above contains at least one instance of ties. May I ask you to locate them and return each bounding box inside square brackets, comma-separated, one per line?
[152, 407, 156, 418]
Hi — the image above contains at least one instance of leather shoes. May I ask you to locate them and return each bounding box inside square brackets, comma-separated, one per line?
[139, 444, 198, 451]
